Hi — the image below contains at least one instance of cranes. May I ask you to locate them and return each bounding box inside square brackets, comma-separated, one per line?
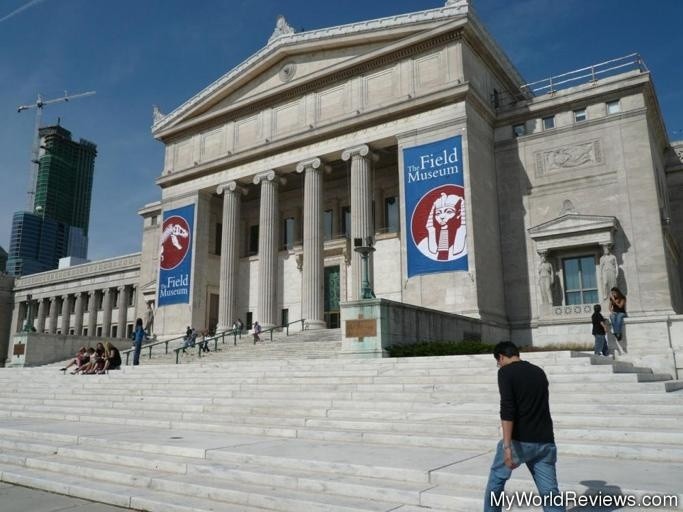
[15, 86, 98, 213]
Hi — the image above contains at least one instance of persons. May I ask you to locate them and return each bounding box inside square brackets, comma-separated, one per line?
[182, 326, 210, 352]
[141, 302, 152, 336]
[484, 340, 565, 511]
[252, 321, 260, 342]
[98, 342, 121, 374]
[608, 287, 626, 341]
[590, 304, 611, 357]
[83, 342, 108, 373]
[60, 346, 89, 375]
[536, 256, 554, 303]
[70, 346, 96, 374]
[237, 318, 242, 339]
[131, 317, 148, 365]
[232, 321, 237, 336]
[599, 246, 618, 299]
